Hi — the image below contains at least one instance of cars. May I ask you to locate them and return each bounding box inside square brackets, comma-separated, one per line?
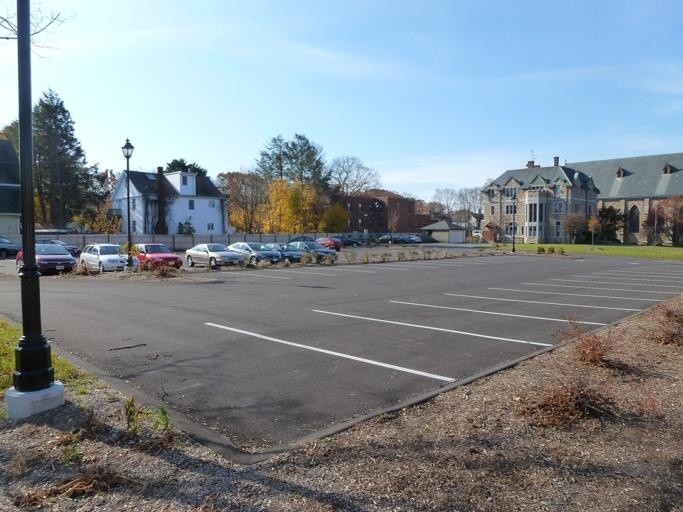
[0, 235, 19, 258]
[35, 239, 79, 255]
[79, 243, 139, 272]
[287, 232, 423, 251]
[15, 244, 76, 273]
[226, 242, 280, 266]
[288, 241, 337, 264]
[184, 243, 245, 269]
[264, 242, 309, 265]
[130, 243, 182, 269]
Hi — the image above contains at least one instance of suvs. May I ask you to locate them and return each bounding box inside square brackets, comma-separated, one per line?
[471, 229, 480, 237]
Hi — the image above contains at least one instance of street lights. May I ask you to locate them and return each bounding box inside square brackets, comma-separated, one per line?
[509, 195, 516, 253]
[121, 137, 135, 272]
